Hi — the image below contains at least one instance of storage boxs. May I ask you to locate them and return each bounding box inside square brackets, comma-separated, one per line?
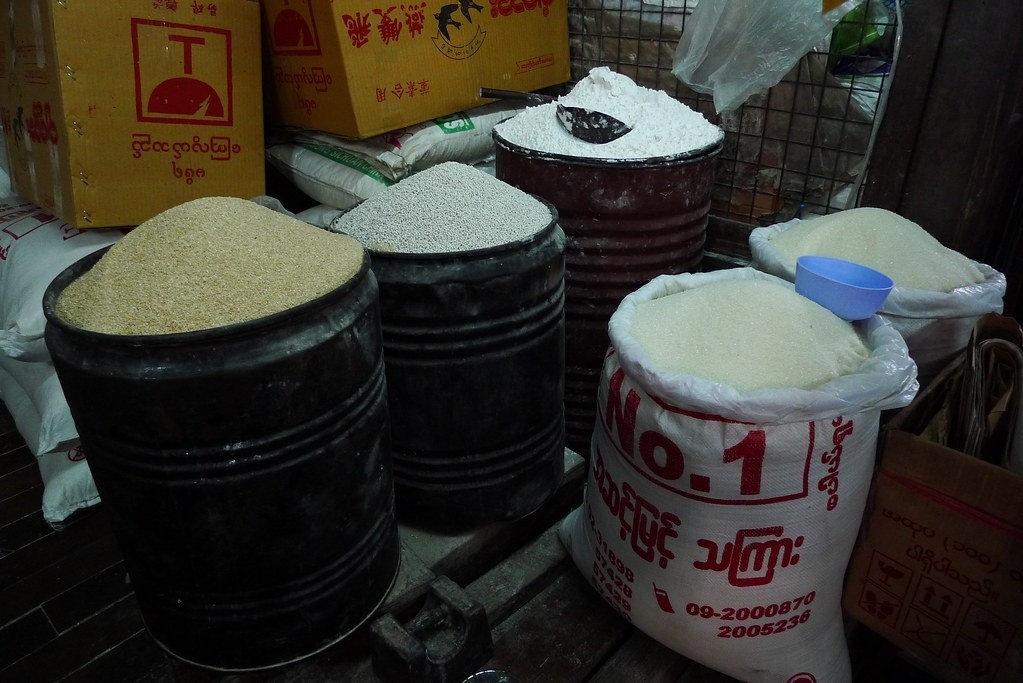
[841, 350, 1023, 683]
[0, 0, 266, 229]
[260, 0, 571, 140]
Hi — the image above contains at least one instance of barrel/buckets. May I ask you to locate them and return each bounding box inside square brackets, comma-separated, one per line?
[41, 222, 401, 673]
[328, 189, 566, 536]
[493, 116, 726, 478]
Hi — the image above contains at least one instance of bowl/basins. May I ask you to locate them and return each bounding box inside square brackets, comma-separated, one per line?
[795, 255, 895, 324]
